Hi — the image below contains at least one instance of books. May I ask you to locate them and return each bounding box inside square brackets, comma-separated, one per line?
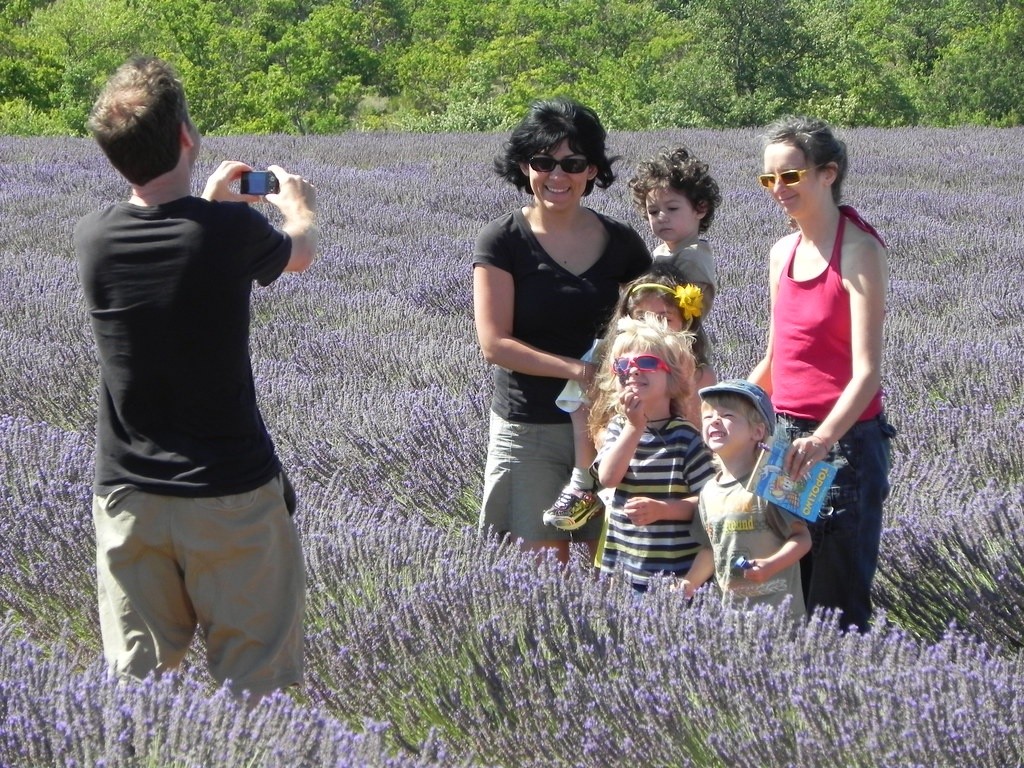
[746, 435, 839, 525]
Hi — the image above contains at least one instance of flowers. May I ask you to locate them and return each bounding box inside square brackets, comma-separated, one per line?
[674, 281, 705, 321]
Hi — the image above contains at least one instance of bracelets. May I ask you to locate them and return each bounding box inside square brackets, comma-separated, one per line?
[812, 434, 829, 455]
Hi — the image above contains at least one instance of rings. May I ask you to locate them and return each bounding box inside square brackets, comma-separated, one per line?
[797, 449, 807, 456]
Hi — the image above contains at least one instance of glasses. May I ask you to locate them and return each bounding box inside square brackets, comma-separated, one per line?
[528, 155, 589, 173]
[612, 354, 670, 374]
[759, 161, 825, 188]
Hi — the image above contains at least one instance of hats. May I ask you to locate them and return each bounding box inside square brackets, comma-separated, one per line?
[697, 378, 775, 437]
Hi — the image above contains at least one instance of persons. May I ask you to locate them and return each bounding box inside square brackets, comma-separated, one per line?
[596, 318, 719, 594]
[471, 102, 656, 569]
[678, 378, 814, 622]
[73, 56, 323, 709]
[587, 273, 721, 580]
[744, 119, 894, 636]
[542, 145, 720, 532]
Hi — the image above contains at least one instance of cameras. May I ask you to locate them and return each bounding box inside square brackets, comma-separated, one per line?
[240, 171, 278, 195]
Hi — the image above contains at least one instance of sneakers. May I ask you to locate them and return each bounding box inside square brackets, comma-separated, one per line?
[542, 479, 605, 531]
